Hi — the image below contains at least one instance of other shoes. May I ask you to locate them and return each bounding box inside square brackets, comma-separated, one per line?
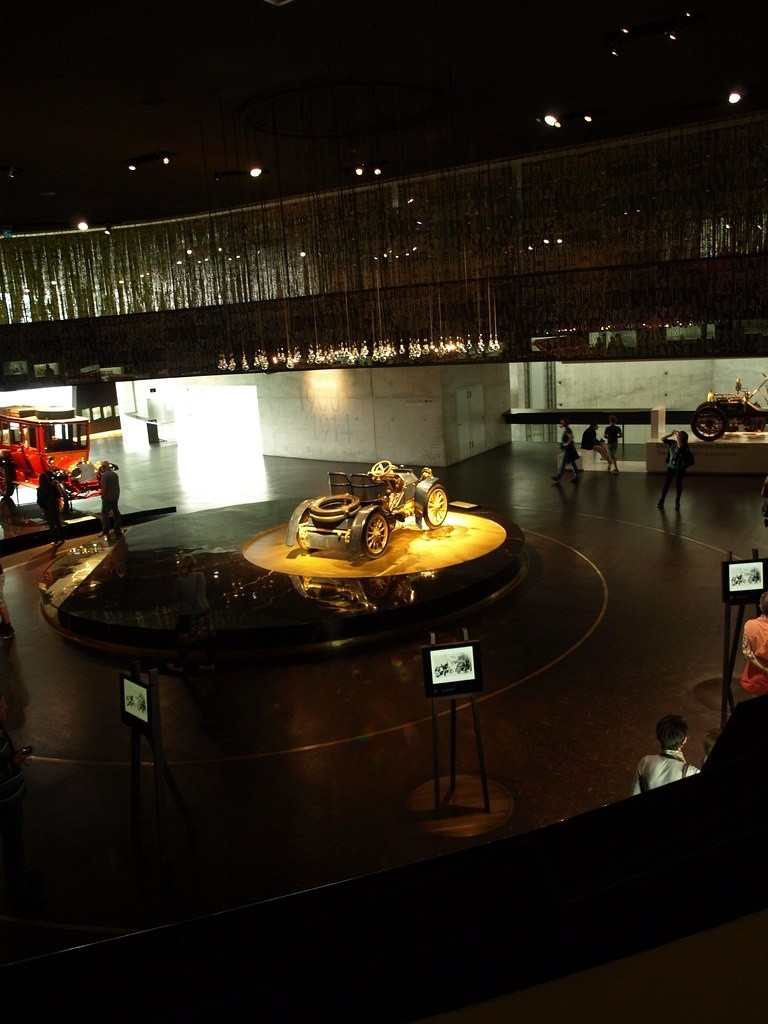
[47, 537, 57, 541]
[98, 531, 111, 537]
[114, 528, 122, 536]
[655, 499, 664, 508]
[612, 469, 619, 473]
[675, 500, 680, 508]
[569, 476, 579, 483]
[56, 537, 64, 541]
[550, 476, 560, 481]
[0, 624, 15, 639]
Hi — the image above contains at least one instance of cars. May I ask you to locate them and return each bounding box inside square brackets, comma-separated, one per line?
[690, 372, 768, 442]
[286, 460, 449, 561]
[0, 400, 120, 514]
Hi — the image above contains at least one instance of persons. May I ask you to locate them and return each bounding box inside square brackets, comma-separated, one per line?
[0, 451, 19, 511]
[166, 554, 220, 671]
[595, 333, 624, 357]
[550, 418, 580, 483]
[0, 563, 16, 639]
[628, 715, 699, 798]
[656, 430, 695, 511]
[604, 415, 623, 473]
[740, 592, 768, 697]
[98, 461, 123, 539]
[36, 473, 66, 545]
[45, 364, 55, 377]
[580, 421, 612, 465]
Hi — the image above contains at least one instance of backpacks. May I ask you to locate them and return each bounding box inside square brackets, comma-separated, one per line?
[684, 451, 696, 468]
[43, 485, 60, 510]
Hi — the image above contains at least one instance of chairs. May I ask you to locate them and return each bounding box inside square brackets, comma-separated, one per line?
[328, 471, 386, 501]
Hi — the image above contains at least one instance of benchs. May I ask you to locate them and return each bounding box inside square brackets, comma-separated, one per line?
[558, 444, 600, 470]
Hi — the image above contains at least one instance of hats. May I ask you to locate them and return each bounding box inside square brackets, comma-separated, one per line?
[676, 430, 688, 442]
